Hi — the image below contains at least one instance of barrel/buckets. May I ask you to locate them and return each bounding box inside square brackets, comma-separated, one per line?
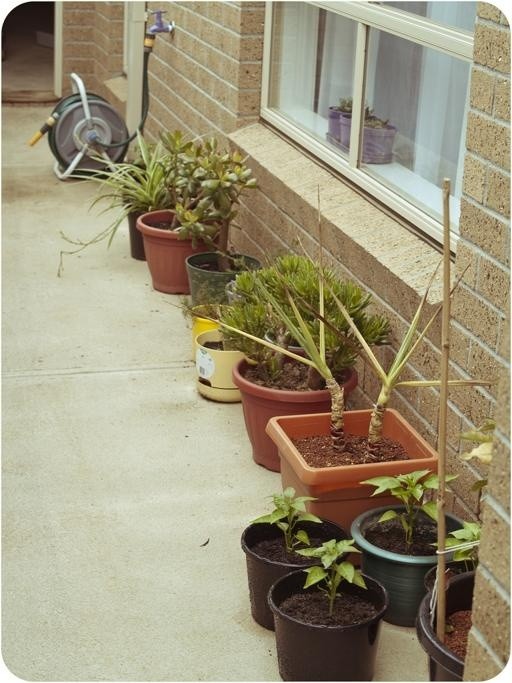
[50, 93, 129, 180]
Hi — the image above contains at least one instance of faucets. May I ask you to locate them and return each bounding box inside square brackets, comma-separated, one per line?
[145, 10, 176, 33]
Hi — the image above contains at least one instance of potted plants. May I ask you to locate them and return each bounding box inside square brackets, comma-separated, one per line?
[57, 129, 176, 277]
[133, 131, 230, 296]
[327, 97, 396, 165]
[191, 284, 247, 402]
[265, 537, 390, 681]
[224, 257, 394, 472]
[176, 139, 265, 306]
[238, 486, 353, 630]
[394, 356, 497, 681]
[186, 177, 474, 535]
[350, 462, 474, 628]
[182, 282, 224, 347]
